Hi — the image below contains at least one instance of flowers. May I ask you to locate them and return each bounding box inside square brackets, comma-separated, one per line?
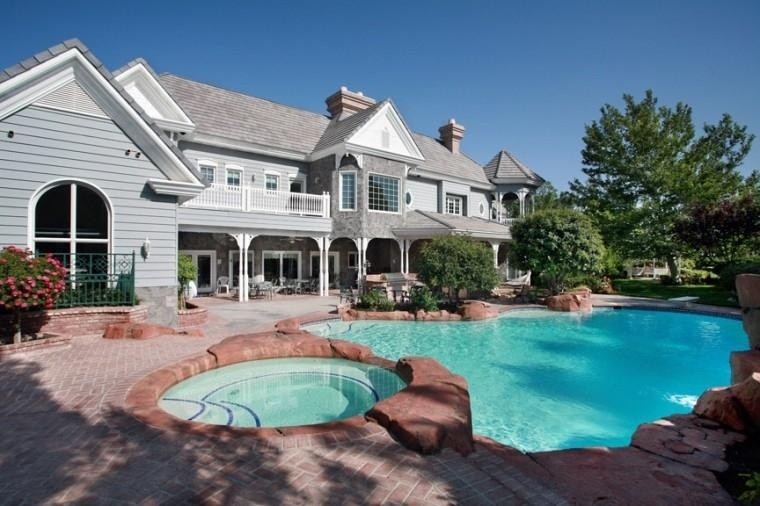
[0, 244, 69, 319]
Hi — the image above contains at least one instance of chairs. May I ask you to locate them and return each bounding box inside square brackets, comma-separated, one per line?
[340, 285, 423, 302]
[216, 274, 319, 301]
